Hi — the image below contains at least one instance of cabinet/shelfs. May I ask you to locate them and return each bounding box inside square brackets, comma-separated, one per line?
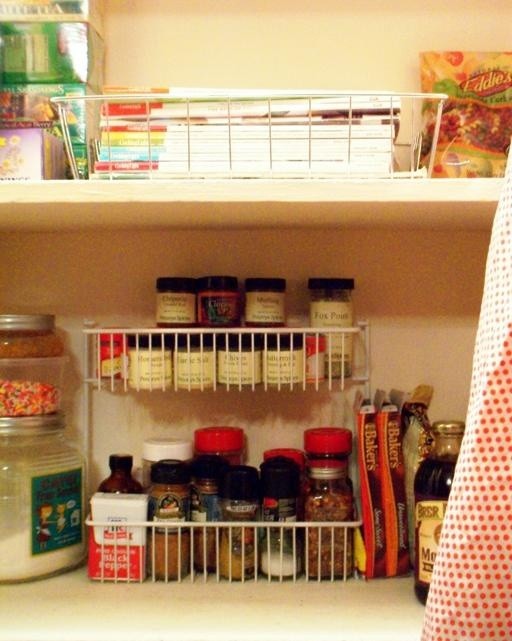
[3, 179, 501, 641]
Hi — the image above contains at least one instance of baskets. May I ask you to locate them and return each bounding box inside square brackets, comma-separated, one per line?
[50, 93, 449, 180]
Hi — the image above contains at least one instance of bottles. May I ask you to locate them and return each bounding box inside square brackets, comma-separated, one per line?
[300, 464, 354, 580]
[0, 313, 89, 583]
[411, 417, 466, 610]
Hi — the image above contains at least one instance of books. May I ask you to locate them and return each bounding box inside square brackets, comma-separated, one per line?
[89, 82, 426, 179]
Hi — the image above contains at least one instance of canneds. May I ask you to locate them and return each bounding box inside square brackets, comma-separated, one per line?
[0, 313, 63, 381]
[0, 412, 87, 584]
[99, 328, 326, 387]
[154, 275, 288, 328]
[308, 274, 356, 383]
